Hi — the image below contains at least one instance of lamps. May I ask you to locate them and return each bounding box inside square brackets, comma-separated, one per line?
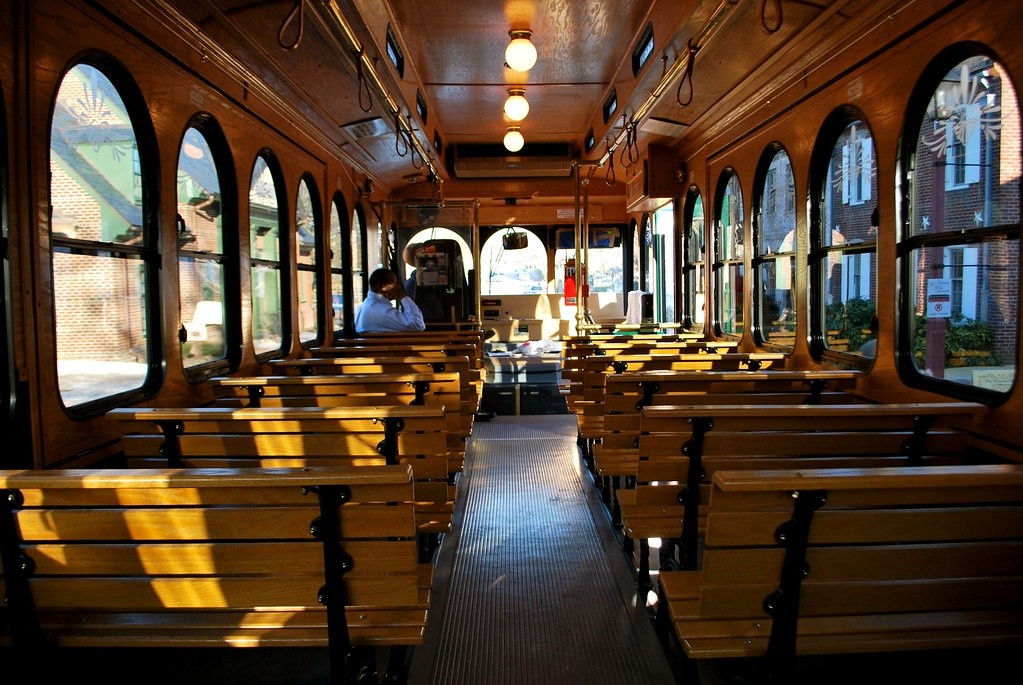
[503, 126, 524, 152]
[503, 88, 529, 122]
[505, 30, 537, 72]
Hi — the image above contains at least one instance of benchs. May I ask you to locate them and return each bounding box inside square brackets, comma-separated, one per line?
[307, 344, 484, 415]
[337, 336, 486, 384]
[266, 355, 475, 442]
[566, 341, 739, 471]
[592, 367, 864, 557]
[0, 463, 436, 685]
[576, 352, 786, 502]
[105, 407, 458, 565]
[358, 330, 489, 375]
[556, 334, 710, 451]
[654, 466, 1023, 685]
[615, 402, 990, 638]
[206, 376, 468, 486]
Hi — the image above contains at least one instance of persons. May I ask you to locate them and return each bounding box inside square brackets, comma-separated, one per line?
[355, 269, 426, 334]
[402, 243, 423, 299]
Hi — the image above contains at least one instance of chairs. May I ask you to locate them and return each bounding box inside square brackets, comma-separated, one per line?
[414, 239, 464, 329]
